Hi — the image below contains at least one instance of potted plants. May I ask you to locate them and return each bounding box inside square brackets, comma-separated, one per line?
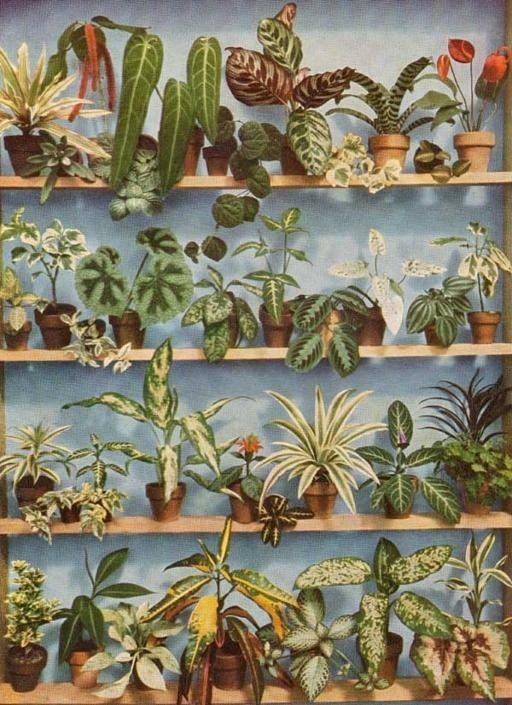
[433, 220, 510, 344]
[53, 547, 156, 686]
[76, 229, 195, 348]
[5, 43, 108, 177]
[235, 2, 353, 175]
[81, 599, 183, 699]
[285, 284, 377, 376]
[330, 226, 446, 346]
[281, 587, 360, 701]
[413, 529, 512, 705]
[0, 560, 62, 693]
[203, 106, 232, 175]
[0, 423, 75, 519]
[325, 56, 457, 175]
[183, 193, 260, 363]
[1, 206, 89, 350]
[2, 263, 39, 351]
[227, 432, 280, 523]
[416, 367, 512, 514]
[53, 486, 82, 522]
[108, 31, 221, 190]
[293, 536, 454, 687]
[253, 385, 388, 516]
[68, 433, 141, 523]
[357, 401, 461, 526]
[438, 37, 510, 173]
[61, 339, 256, 521]
[407, 275, 476, 347]
[145, 516, 300, 703]
[233, 208, 315, 347]
[58, 14, 151, 159]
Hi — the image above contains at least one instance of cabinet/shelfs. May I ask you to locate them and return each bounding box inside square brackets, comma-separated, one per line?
[4, 170, 512, 702]
